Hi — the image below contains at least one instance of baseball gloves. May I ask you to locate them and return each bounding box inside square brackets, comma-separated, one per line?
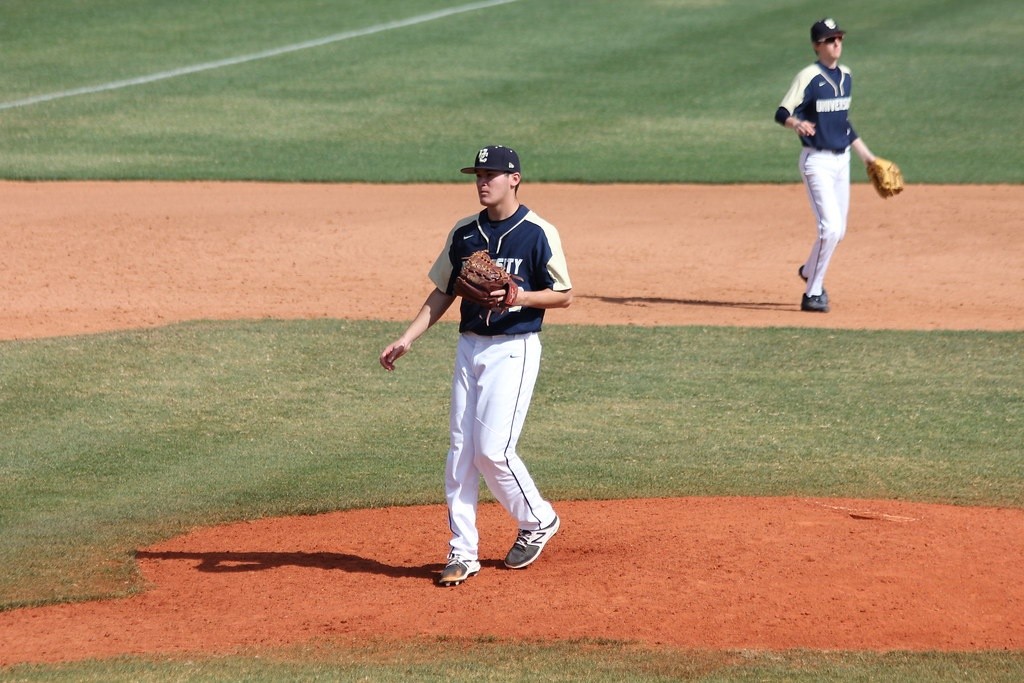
[454, 250, 525, 327]
[866, 157, 904, 200]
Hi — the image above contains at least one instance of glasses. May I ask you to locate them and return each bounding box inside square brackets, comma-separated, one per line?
[820, 35, 844, 43]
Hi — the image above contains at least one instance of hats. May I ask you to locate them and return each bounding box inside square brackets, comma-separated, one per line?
[811, 17, 846, 44]
[461, 146, 521, 173]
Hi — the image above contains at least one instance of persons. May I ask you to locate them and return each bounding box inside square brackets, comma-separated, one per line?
[773, 15, 877, 312]
[378, 144, 576, 587]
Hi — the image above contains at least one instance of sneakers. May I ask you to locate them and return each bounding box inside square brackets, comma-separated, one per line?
[440, 560, 481, 586]
[797, 265, 828, 304]
[505, 516, 561, 569]
[801, 293, 828, 312]
